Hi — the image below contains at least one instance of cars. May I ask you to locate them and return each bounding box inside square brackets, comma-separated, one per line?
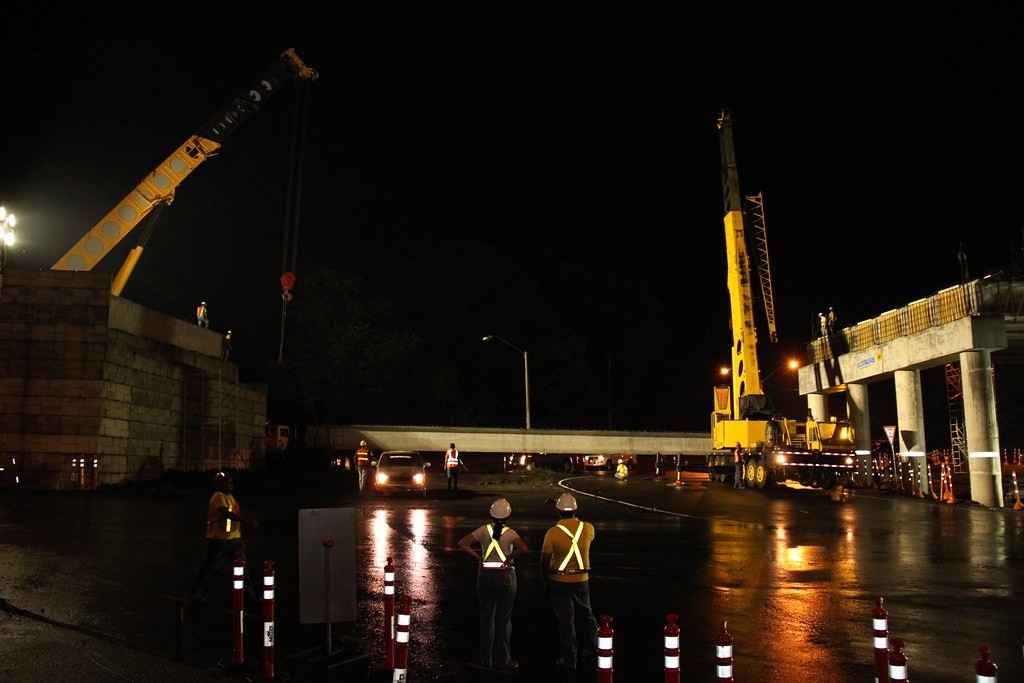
[371, 450, 431, 498]
[502, 452, 535, 473]
[584, 454, 637, 470]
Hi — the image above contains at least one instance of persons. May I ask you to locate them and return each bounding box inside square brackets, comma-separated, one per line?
[457, 498, 527, 671]
[540, 496, 598, 674]
[444, 444, 467, 495]
[197, 302, 209, 328]
[226, 330, 233, 360]
[819, 313, 828, 336]
[734, 443, 747, 490]
[615, 459, 628, 480]
[829, 306, 837, 333]
[192, 472, 264, 617]
[354, 440, 377, 493]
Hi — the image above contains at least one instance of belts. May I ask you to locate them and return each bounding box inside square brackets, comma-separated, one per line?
[546, 568, 589, 574]
[479, 562, 515, 566]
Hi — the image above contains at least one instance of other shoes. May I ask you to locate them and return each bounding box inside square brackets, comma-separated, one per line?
[733, 485, 745, 490]
[623, 478, 628, 481]
[362, 487, 367, 491]
[246, 589, 256, 600]
[505, 660, 519, 669]
[191, 592, 205, 602]
[448, 490, 457, 492]
[557, 658, 576, 669]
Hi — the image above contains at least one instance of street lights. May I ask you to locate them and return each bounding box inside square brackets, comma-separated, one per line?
[481, 334, 530, 431]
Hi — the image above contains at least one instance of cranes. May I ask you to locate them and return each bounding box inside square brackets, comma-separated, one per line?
[46, 46, 320, 304]
[709, 100, 858, 491]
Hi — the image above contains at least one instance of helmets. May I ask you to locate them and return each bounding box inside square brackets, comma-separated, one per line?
[618, 458, 623, 463]
[215, 471, 234, 486]
[359, 440, 368, 447]
[450, 443, 456, 448]
[489, 498, 511, 519]
[556, 493, 578, 511]
[736, 442, 741, 446]
